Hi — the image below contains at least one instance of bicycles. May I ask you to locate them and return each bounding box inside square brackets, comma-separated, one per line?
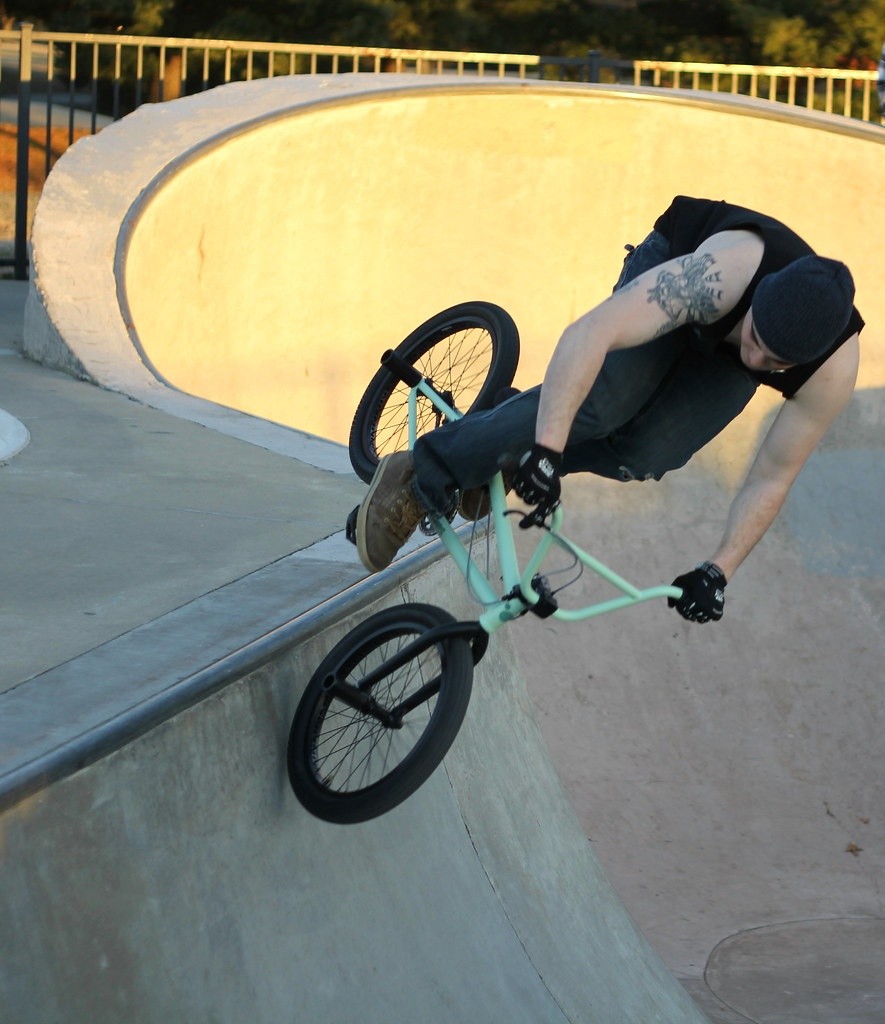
[288, 301, 684, 824]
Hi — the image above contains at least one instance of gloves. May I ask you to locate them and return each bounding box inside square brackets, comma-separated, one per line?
[664, 564, 728, 624]
[512, 445, 563, 508]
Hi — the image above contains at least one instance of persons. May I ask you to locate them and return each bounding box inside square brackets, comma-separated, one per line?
[356, 195, 865, 623]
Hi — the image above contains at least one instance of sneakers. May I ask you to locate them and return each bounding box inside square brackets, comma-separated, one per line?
[355, 450, 421, 576]
[453, 478, 495, 525]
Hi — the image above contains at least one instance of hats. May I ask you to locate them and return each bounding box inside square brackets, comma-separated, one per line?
[750, 257, 858, 366]
